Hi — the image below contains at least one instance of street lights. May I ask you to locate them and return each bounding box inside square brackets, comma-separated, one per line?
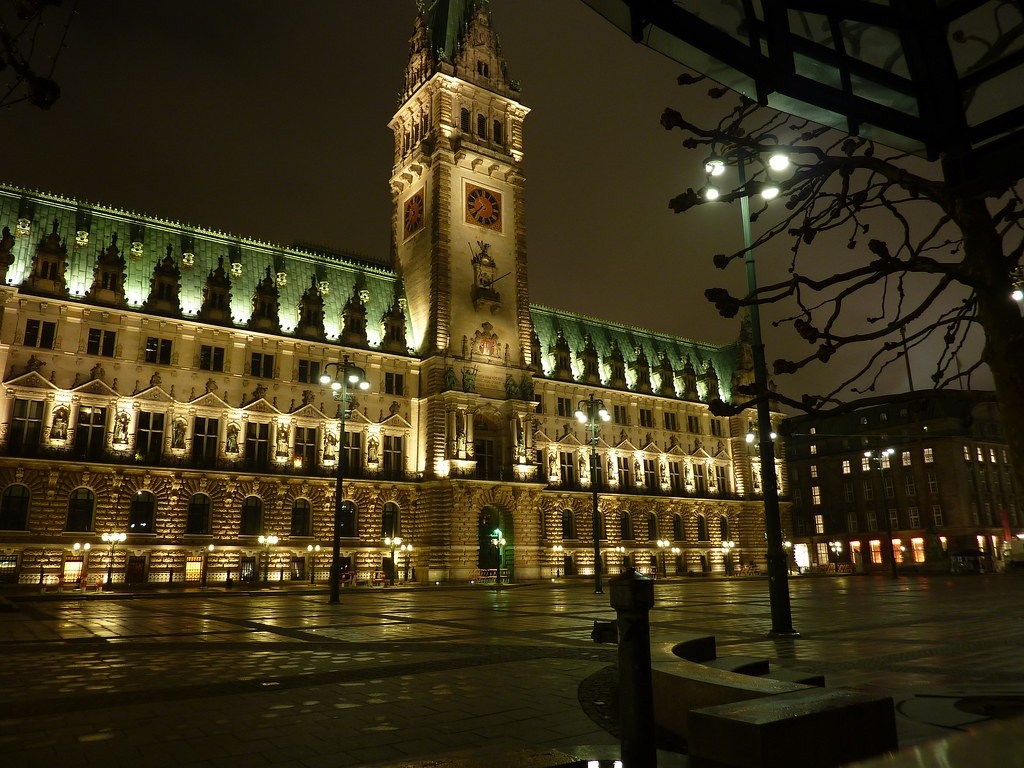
[553, 544, 563, 578]
[574, 392, 608, 594]
[308, 544, 320, 585]
[616, 546, 625, 574]
[863, 446, 901, 581]
[384, 536, 403, 587]
[657, 539, 670, 579]
[491, 528, 507, 583]
[102, 532, 127, 591]
[703, 152, 806, 638]
[721, 540, 735, 577]
[257, 535, 279, 583]
[319, 352, 371, 604]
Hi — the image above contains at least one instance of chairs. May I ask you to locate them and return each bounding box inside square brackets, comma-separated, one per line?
[732, 565, 762, 577]
[634, 565, 662, 579]
[467, 569, 511, 583]
[341, 571, 385, 587]
[804, 563, 853, 573]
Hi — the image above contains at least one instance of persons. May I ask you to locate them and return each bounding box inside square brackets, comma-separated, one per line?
[457, 432, 468, 458]
[505, 373, 534, 401]
[445, 366, 476, 393]
[50, 409, 378, 463]
[517, 432, 716, 487]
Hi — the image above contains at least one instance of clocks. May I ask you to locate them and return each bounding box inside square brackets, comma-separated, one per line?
[462, 178, 505, 237]
[401, 180, 426, 244]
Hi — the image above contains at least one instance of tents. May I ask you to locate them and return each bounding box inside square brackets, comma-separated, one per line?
[948, 541, 987, 574]
